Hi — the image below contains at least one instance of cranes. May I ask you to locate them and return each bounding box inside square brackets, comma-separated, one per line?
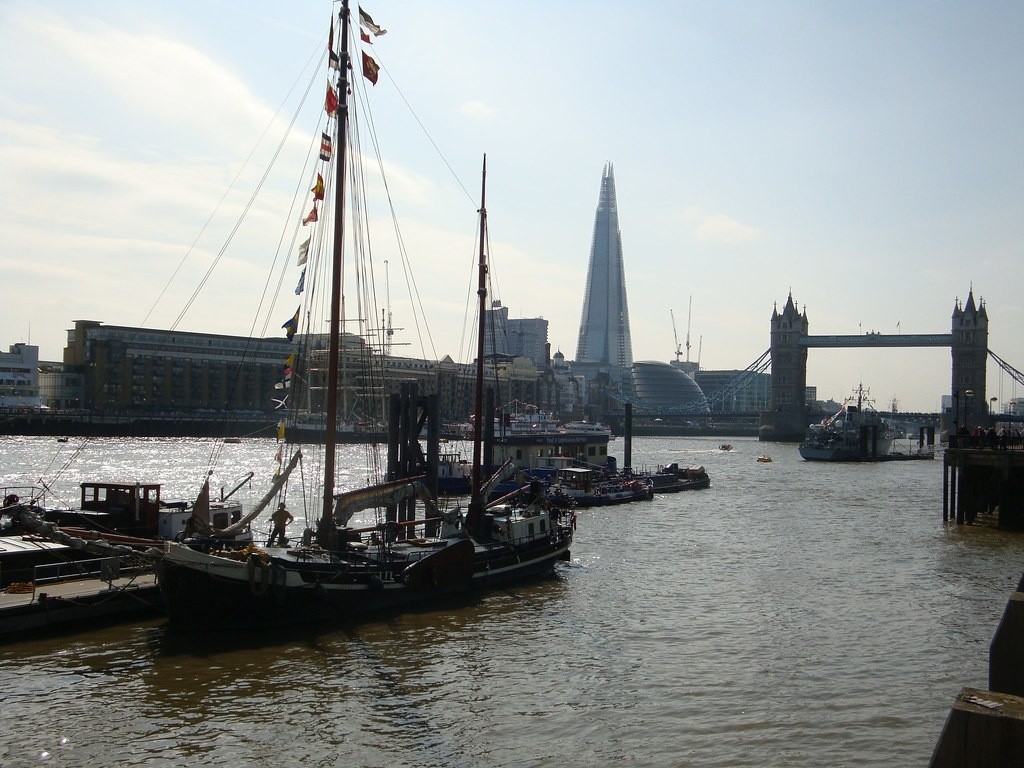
[669, 309, 683, 362]
[686, 295, 691, 362]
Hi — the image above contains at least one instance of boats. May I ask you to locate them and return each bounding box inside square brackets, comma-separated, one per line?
[649, 462, 710, 493]
[523, 468, 654, 501]
[224, 439, 241, 443]
[719, 444, 733, 452]
[757, 455, 772, 462]
[466, 400, 612, 443]
[797, 381, 895, 461]
[0, 482, 253, 583]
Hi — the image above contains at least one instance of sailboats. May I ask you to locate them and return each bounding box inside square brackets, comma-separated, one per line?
[162, 1, 573, 614]
[388, 149, 614, 497]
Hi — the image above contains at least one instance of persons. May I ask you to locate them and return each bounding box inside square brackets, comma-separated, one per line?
[958, 424, 1024, 449]
[264, 502, 293, 547]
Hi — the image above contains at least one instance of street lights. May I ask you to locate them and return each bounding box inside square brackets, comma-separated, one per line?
[1009, 401, 1017, 430]
[989, 396, 997, 428]
[963, 389, 974, 426]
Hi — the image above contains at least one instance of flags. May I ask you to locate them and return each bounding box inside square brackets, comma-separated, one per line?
[295, 267, 306, 295]
[281, 305, 300, 343]
[324, 80, 338, 120]
[310, 174, 324, 202]
[361, 51, 380, 86]
[358, 6, 388, 44]
[296, 238, 311, 266]
[319, 132, 331, 161]
[272, 351, 295, 491]
[328, 15, 340, 71]
[303, 202, 318, 225]
